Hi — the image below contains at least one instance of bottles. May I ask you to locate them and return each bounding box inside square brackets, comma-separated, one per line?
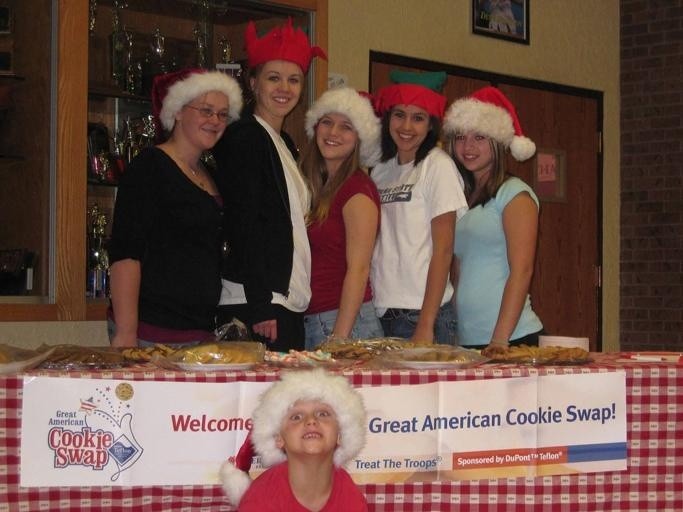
[89, 204, 108, 298]
[119, 117, 142, 170]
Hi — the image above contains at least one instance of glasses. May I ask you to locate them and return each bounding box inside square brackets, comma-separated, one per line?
[183, 105, 233, 123]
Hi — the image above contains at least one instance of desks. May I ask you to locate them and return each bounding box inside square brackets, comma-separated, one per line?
[1, 343, 683, 508]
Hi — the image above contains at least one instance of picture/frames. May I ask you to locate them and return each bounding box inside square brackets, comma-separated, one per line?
[472, 0, 531, 45]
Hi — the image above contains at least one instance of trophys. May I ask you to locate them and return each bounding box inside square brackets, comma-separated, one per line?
[88, 1, 242, 299]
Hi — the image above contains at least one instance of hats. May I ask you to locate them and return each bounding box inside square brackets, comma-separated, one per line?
[443, 86, 536, 163]
[376, 83, 448, 120]
[305, 87, 384, 168]
[150, 69, 244, 132]
[218, 368, 369, 507]
[244, 16, 328, 76]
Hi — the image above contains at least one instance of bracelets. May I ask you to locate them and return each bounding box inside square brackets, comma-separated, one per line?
[490, 338, 510, 346]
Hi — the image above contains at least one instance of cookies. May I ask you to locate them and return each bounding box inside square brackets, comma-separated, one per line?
[115, 383, 134, 401]
[0, 338, 264, 376]
[313, 338, 592, 369]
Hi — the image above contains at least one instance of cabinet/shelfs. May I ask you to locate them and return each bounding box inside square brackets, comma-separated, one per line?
[0, 1, 329, 323]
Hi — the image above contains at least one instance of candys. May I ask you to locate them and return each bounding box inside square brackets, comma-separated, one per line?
[263, 348, 336, 368]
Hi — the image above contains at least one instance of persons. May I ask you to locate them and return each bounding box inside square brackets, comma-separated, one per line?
[369, 82, 458, 349]
[212, 15, 328, 352]
[108, 67, 244, 348]
[236, 366, 370, 512]
[297, 86, 386, 353]
[442, 84, 547, 348]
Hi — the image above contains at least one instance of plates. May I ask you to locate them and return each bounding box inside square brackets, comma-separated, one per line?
[489, 357, 594, 368]
[42, 361, 134, 369]
[384, 359, 460, 371]
[0, 344, 56, 375]
[172, 357, 252, 374]
[264, 359, 354, 371]
